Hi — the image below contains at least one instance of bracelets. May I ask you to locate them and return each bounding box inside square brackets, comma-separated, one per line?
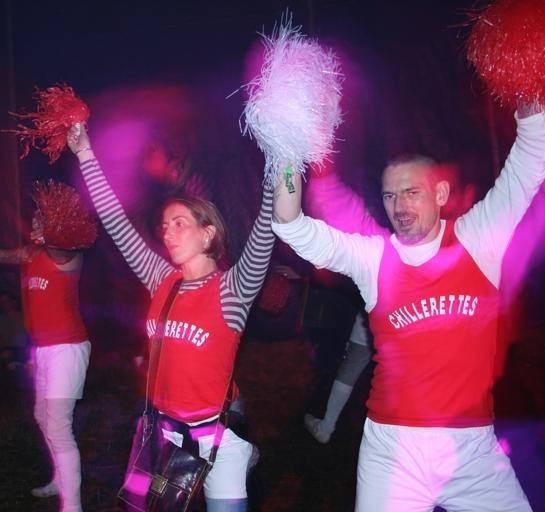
[76, 147, 93, 154]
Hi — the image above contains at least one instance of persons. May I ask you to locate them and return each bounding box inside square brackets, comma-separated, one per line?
[1, 193, 91, 512]
[304, 312, 372, 444]
[273, 265, 327, 292]
[68, 122, 276, 512]
[271, 95, 545, 512]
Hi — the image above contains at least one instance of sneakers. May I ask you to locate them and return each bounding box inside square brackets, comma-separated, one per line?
[304, 412, 331, 444]
[32, 481, 59, 498]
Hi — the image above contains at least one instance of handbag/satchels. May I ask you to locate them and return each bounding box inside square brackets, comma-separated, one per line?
[115, 432, 213, 512]
